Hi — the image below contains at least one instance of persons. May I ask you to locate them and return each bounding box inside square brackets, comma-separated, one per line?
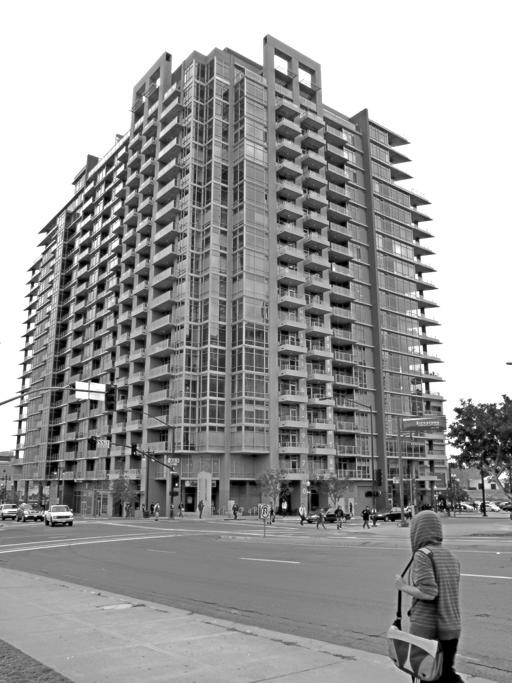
[393, 508, 464, 682]
[125, 496, 412, 529]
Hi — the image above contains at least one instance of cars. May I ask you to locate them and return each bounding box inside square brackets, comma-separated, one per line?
[0, 504, 19, 520]
[458, 498, 512, 511]
[306, 507, 351, 524]
[15, 502, 46, 521]
[371, 505, 411, 520]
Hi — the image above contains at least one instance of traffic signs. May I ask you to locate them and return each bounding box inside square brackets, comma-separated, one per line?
[96, 439, 109, 447]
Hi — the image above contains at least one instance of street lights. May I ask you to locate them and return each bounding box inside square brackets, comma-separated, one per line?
[505, 360, 512, 366]
[118, 406, 177, 518]
[317, 394, 378, 528]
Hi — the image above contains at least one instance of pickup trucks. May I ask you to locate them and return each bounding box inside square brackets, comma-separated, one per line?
[43, 503, 74, 526]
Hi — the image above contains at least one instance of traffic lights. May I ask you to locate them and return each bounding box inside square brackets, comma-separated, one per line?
[130, 442, 137, 455]
[173, 474, 179, 488]
[106, 384, 116, 411]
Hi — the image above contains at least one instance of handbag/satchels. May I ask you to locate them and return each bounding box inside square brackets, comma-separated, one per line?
[387, 625, 444, 681]
